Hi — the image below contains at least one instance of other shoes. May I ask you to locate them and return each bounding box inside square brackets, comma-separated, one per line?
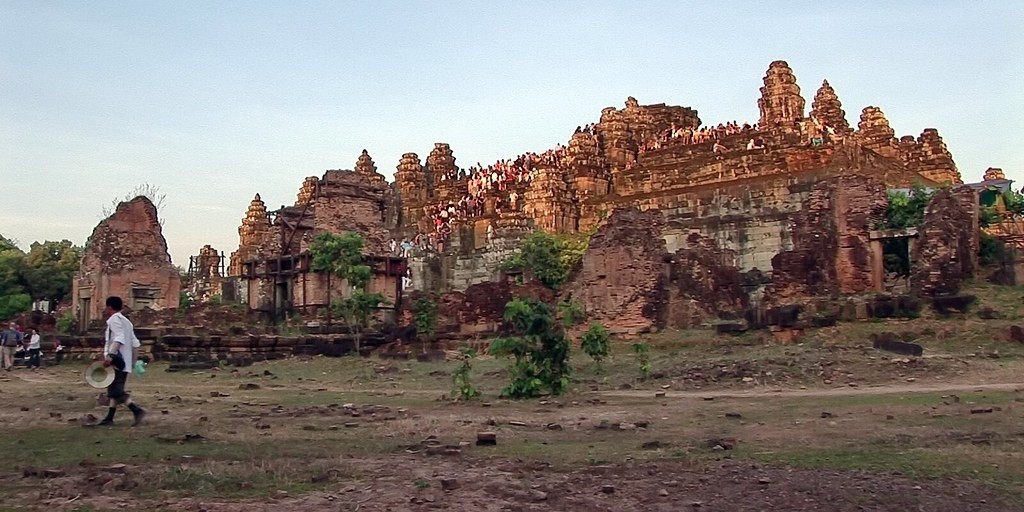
[131, 408, 146, 426]
[98, 419, 114, 425]
[5, 364, 12, 370]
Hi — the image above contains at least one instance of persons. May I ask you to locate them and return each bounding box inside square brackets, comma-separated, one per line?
[95, 296, 145, 429]
[0, 321, 41, 371]
[388, 121, 756, 288]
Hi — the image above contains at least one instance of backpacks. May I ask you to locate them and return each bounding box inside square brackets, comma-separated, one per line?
[5, 330, 17, 346]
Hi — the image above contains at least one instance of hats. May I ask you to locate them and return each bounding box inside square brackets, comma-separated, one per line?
[85, 361, 115, 388]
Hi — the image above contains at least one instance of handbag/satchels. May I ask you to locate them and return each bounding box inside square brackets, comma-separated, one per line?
[27, 346, 30, 351]
[108, 350, 126, 369]
